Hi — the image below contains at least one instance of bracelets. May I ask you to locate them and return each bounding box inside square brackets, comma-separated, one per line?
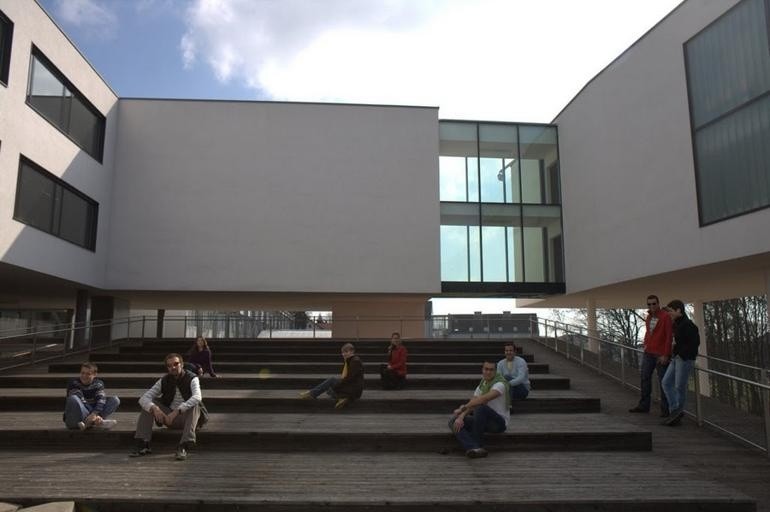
[460, 404, 467, 412]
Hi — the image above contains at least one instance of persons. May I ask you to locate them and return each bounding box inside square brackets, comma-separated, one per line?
[379, 332, 408, 390]
[128, 353, 209, 461]
[496, 342, 531, 415]
[447, 359, 511, 458]
[61, 361, 120, 431]
[661, 300, 700, 426]
[628, 294, 671, 419]
[181, 336, 223, 380]
[298, 343, 363, 410]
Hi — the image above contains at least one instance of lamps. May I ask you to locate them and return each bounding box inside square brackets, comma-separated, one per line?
[498, 166, 509, 181]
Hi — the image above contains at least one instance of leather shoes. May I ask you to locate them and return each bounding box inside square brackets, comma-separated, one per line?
[128, 446, 151, 457]
[175, 446, 192, 460]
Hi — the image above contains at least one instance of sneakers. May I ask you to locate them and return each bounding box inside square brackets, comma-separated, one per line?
[96, 419, 117, 429]
[660, 404, 686, 425]
[627, 406, 648, 413]
[465, 447, 488, 457]
[297, 391, 314, 401]
[335, 398, 346, 408]
[78, 419, 87, 431]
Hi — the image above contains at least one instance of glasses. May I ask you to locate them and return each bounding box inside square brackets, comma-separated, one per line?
[647, 303, 657, 305]
[166, 361, 180, 367]
